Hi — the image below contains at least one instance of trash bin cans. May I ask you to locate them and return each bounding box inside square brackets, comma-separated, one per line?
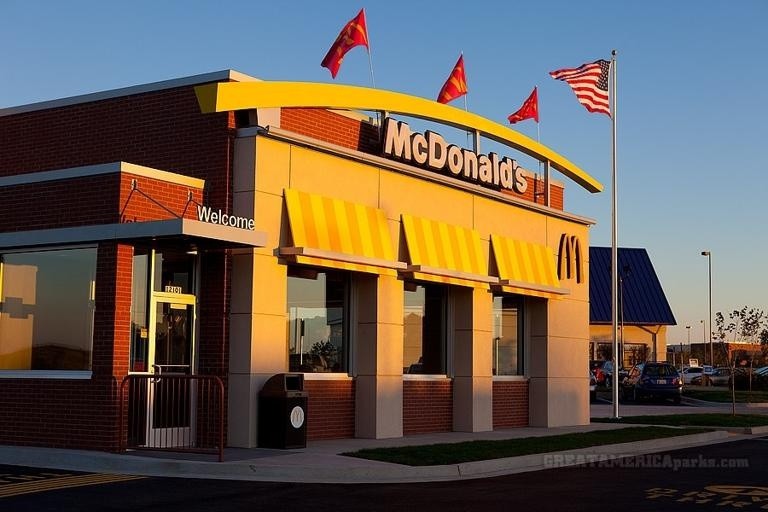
[257, 373, 309, 449]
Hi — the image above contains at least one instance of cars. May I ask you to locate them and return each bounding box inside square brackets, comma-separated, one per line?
[588, 359, 766, 402]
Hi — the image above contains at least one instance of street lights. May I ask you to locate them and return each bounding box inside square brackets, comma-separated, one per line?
[686, 325, 691, 357]
[700, 252, 714, 368]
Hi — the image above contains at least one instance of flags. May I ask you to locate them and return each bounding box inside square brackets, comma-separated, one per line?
[548, 60, 614, 122]
[437, 54, 468, 105]
[319, 7, 370, 82]
[508, 87, 539, 125]
